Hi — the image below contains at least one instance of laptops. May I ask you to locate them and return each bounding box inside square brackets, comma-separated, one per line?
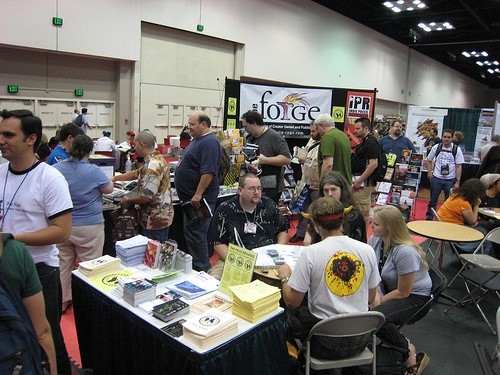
[234, 227, 276, 268]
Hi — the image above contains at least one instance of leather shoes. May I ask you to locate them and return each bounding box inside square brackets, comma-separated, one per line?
[289, 234, 304, 242]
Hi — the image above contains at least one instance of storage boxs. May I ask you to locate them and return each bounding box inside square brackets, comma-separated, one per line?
[227, 128, 245, 177]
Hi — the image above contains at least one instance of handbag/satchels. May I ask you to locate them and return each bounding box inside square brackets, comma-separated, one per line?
[288, 180, 310, 213]
[260, 175, 276, 188]
[110, 207, 140, 242]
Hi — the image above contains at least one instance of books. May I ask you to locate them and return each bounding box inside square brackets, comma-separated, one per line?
[242, 142, 259, 160]
[78, 234, 282, 350]
[371, 149, 423, 223]
[102, 180, 137, 211]
[293, 146, 308, 164]
[181, 197, 214, 227]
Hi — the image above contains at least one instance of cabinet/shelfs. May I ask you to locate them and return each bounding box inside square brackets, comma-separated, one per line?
[371, 153, 424, 220]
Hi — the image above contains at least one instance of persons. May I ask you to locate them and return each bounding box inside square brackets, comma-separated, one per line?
[289, 112, 416, 243]
[476, 135, 500, 232]
[174, 110, 222, 272]
[114, 128, 160, 171]
[111, 131, 174, 243]
[180, 126, 192, 150]
[35, 128, 61, 162]
[451, 131, 465, 153]
[93, 131, 117, 155]
[50, 134, 114, 315]
[72, 108, 93, 134]
[303, 171, 367, 246]
[279, 197, 381, 375]
[424, 127, 441, 157]
[0, 109, 74, 375]
[425, 129, 465, 221]
[207, 172, 289, 281]
[437, 179, 487, 269]
[0, 232, 57, 375]
[367, 205, 432, 375]
[241, 110, 291, 205]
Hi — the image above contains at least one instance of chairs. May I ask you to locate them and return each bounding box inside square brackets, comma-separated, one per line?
[426, 207, 469, 271]
[381, 262, 447, 357]
[447, 227, 500, 335]
[295, 311, 386, 375]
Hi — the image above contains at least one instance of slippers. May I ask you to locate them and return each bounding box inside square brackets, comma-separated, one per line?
[62, 300, 72, 314]
[403, 351, 430, 375]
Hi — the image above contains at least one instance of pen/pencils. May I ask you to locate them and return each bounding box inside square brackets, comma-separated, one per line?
[272, 269, 278, 277]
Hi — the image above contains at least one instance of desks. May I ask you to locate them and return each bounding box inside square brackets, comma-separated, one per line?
[98, 181, 240, 214]
[477, 207, 500, 220]
[406, 220, 484, 306]
[250, 244, 313, 280]
[70, 259, 288, 375]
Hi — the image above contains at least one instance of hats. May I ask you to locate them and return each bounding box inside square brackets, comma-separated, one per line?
[126, 131, 135, 136]
[479, 174, 500, 188]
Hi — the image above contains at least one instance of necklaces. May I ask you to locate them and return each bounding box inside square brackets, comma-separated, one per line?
[241, 205, 257, 234]
[440, 147, 453, 175]
[1, 156, 37, 232]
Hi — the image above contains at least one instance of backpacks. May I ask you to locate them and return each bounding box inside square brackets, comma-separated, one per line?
[75, 114, 85, 127]
[218, 146, 230, 185]
[0, 232, 50, 375]
[364, 137, 388, 182]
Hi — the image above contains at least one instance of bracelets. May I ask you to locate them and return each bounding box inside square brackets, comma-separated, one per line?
[281, 277, 289, 284]
